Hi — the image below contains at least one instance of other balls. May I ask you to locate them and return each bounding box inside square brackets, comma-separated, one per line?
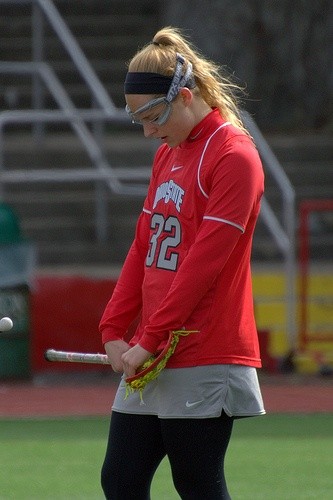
[0, 317, 14, 331]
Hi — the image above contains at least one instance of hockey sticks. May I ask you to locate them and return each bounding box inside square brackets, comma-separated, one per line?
[45, 330, 201, 406]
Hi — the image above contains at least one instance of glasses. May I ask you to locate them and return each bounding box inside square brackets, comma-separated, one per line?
[123, 96, 170, 127]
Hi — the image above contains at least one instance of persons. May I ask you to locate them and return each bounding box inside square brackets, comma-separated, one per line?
[100, 22, 264, 500]
